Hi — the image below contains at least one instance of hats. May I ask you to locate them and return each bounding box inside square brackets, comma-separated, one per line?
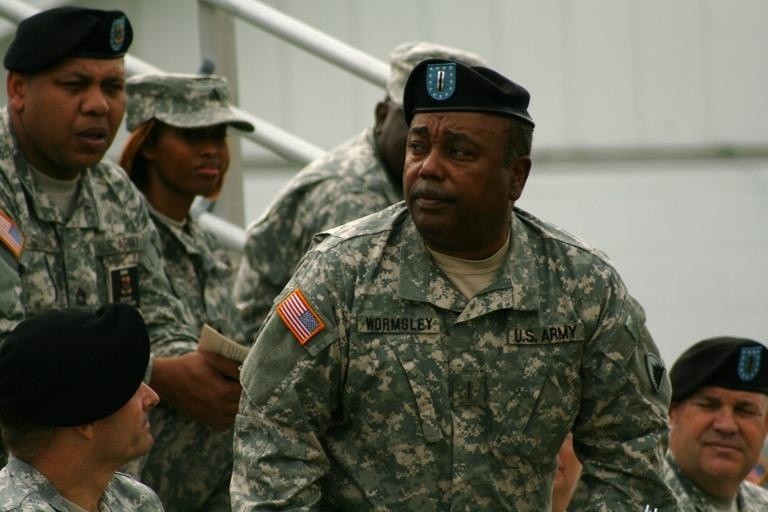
[385, 42, 488, 107]
[4, 5, 133, 78]
[670, 337, 768, 411]
[0, 302, 150, 426]
[125, 74, 256, 133]
[402, 57, 536, 128]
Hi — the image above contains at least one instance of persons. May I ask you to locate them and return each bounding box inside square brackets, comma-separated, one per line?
[550, 430, 584, 510]
[665, 337, 768, 512]
[0, 7, 243, 512]
[0, 303, 163, 512]
[228, 56, 672, 512]
[232, 38, 488, 346]
[119, 72, 253, 341]
